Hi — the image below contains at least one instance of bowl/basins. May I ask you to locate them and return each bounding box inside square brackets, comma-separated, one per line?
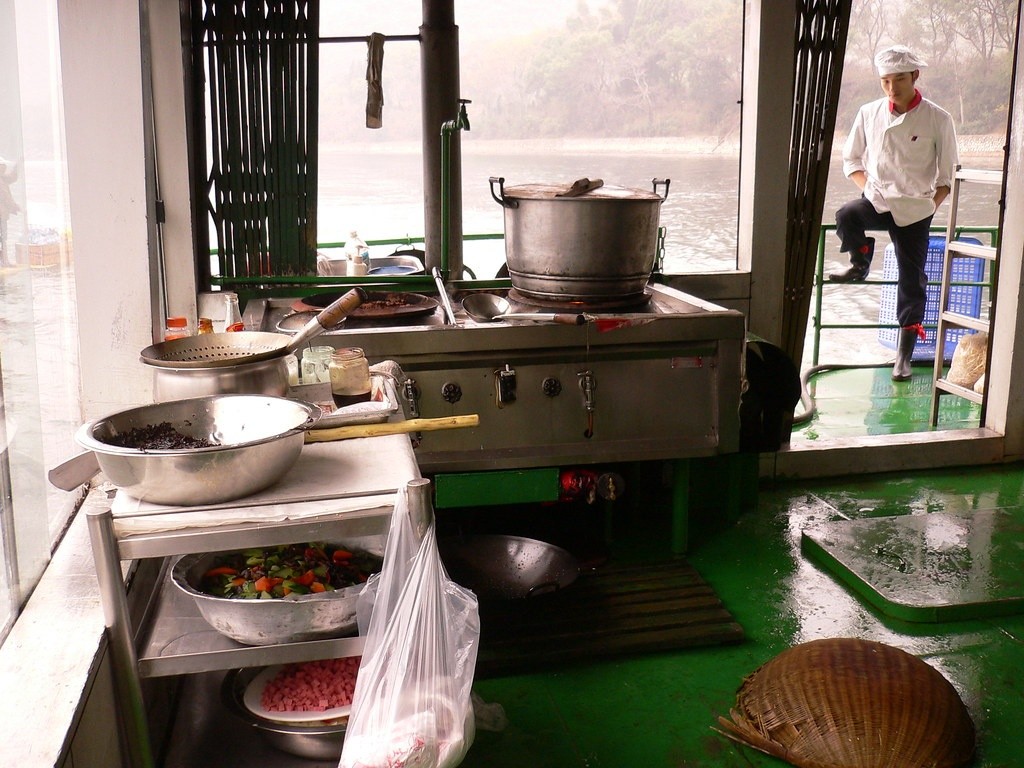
[75, 394, 322, 506]
[220, 666, 347, 762]
[169, 535, 388, 646]
[139, 353, 289, 403]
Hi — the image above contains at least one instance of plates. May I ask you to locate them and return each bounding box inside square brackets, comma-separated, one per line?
[244, 665, 352, 722]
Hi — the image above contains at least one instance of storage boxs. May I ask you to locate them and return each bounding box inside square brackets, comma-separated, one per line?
[876, 236, 986, 363]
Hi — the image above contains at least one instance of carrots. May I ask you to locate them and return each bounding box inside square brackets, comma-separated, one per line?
[205, 546, 370, 597]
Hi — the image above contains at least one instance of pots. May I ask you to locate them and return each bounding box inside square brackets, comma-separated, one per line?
[440, 533, 584, 628]
[490, 175, 671, 303]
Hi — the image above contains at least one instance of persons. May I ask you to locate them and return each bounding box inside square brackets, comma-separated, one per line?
[829, 45, 958, 382]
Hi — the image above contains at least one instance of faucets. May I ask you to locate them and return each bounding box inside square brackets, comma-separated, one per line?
[453, 98, 471, 130]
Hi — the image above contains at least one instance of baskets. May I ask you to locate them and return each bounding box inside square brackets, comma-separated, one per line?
[712, 637, 974, 768]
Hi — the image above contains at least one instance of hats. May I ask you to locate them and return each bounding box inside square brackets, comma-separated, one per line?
[874, 44, 928, 75]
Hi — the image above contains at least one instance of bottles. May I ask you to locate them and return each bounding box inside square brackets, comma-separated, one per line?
[344, 232, 372, 277]
[210, 284, 221, 293]
[197, 318, 215, 335]
[224, 294, 244, 332]
[164, 318, 190, 341]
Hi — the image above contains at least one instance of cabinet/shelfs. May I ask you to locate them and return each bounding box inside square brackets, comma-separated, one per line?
[929, 164, 1006, 427]
[86, 365, 434, 768]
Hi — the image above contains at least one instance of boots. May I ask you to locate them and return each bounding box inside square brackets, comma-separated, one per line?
[891, 329, 919, 381]
[829, 237, 875, 283]
